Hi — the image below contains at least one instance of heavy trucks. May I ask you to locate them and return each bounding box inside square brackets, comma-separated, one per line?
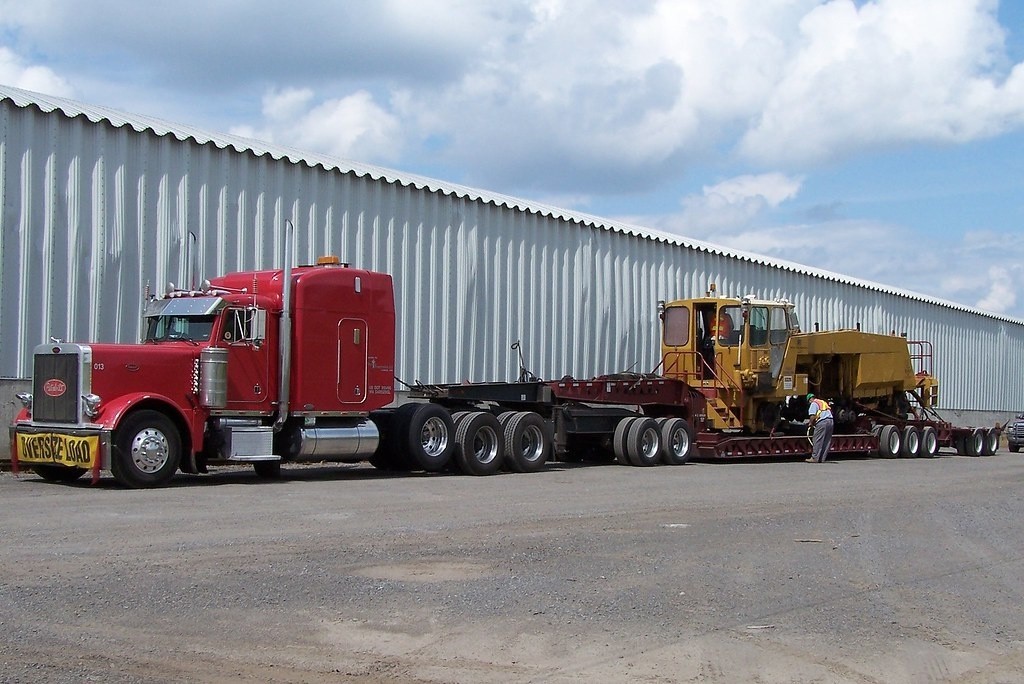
[9, 212, 694, 482]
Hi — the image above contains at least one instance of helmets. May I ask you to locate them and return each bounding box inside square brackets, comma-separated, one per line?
[805, 393, 815, 402]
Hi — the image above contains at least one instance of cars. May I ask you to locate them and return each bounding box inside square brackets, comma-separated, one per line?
[1007, 408, 1024, 454]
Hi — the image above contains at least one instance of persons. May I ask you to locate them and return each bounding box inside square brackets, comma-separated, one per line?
[804, 392, 833, 462]
[708, 306, 733, 350]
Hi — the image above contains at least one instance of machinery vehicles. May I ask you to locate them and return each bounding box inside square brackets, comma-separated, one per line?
[657, 282, 1006, 464]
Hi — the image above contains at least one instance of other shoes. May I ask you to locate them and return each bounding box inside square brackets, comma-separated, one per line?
[805, 458, 819, 463]
